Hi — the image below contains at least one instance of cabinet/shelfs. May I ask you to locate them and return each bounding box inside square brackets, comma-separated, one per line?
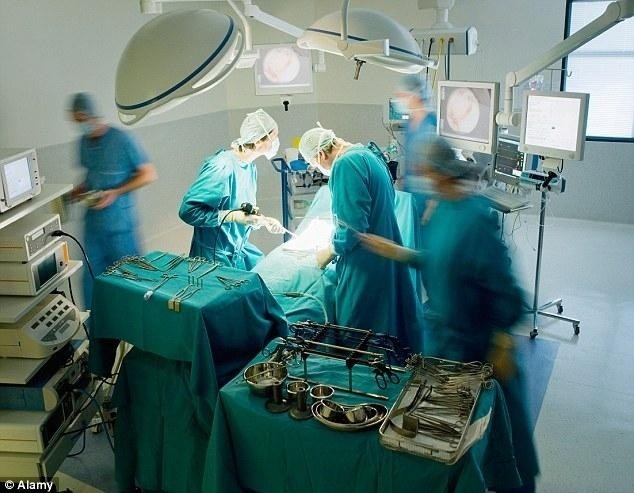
[0, 184, 103, 483]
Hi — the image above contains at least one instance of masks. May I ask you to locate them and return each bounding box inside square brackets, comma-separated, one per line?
[73, 119, 94, 135]
[258, 137, 280, 160]
[316, 155, 337, 177]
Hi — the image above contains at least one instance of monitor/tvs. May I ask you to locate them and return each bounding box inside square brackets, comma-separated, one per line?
[253, 43, 313, 95]
[0, 147, 41, 215]
[518, 90, 589, 161]
[437, 80, 500, 154]
[382, 95, 411, 131]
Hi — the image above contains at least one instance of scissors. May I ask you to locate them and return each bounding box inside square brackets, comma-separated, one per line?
[154, 273, 180, 290]
[215, 276, 251, 290]
[102, 255, 162, 281]
[184, 256, 225, 278]
[408, 361, 496, 448]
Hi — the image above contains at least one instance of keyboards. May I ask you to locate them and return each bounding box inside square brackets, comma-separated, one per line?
[479, 186, 531, 210]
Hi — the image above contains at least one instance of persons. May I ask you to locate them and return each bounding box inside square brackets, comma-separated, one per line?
[178, 108, 286, 273]
[299, 127, 423, 354]
[356, 132, 540, 492]
[64, 92, 158, 326]
[390, 75, 438, 296]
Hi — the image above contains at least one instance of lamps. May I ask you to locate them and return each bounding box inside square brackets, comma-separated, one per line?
[296, 9, 436, 79]
[115, 11, 245, 127]
